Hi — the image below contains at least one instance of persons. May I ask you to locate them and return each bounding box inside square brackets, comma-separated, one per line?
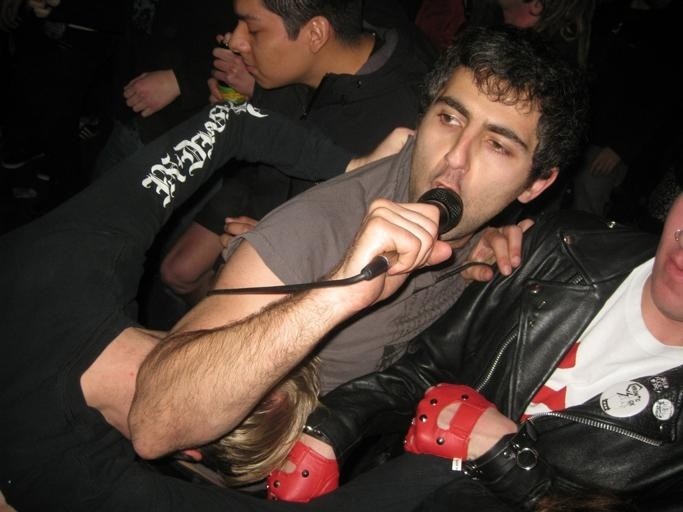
[0, 2, 683, 511]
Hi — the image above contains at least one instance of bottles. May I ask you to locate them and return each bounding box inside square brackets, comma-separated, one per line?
[214, 38, 245, 108]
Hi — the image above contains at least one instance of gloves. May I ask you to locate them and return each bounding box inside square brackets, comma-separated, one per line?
[265, 436, 341, 504]
[404, 378, 497, 462]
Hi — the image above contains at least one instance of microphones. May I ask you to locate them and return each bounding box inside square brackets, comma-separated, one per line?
[362, 189, 462, 280]
